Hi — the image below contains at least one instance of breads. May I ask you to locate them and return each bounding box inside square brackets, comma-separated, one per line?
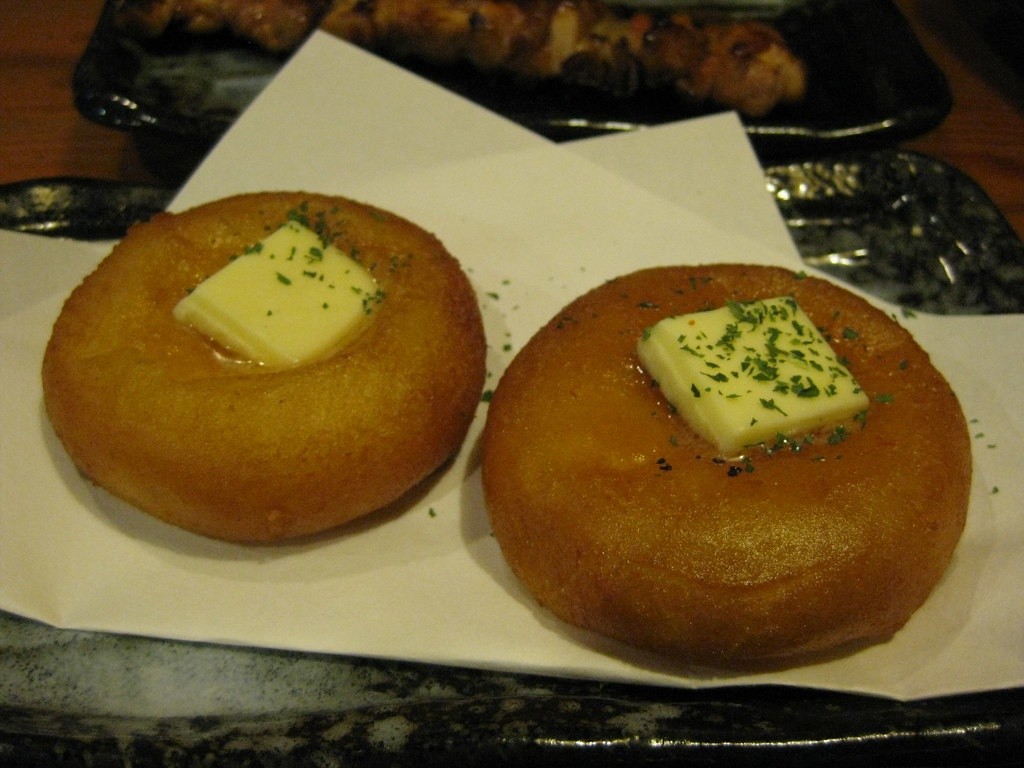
[480, 261, 972, 662]
[42, 189, 490, 543]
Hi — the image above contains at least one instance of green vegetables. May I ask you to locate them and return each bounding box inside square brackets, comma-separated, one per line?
[220, 194, 924, 477]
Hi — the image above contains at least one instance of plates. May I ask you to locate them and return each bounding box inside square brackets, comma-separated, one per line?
[70, 0, 953, 150]
[0, 144, 1024, 768]
